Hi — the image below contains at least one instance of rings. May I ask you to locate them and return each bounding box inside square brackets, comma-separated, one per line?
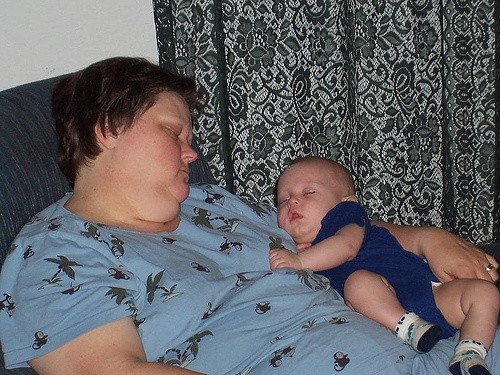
[486, 263, 493, 272]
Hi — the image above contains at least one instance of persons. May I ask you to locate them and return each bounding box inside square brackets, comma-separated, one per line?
[0, 56, 500, 375]
[269, 157, 500, 375]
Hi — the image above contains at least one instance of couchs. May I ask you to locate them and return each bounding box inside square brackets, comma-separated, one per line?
[0, 63, 218, 375]
